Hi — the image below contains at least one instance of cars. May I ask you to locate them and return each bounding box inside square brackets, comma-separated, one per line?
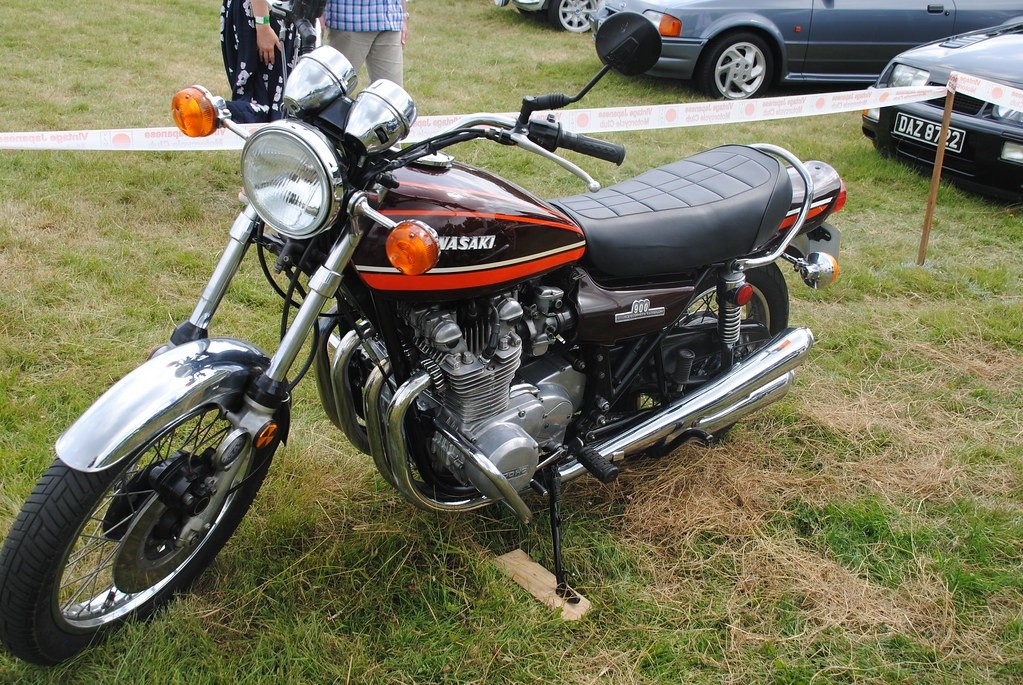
[494, 0, 605, 34]
[861, 16, 1023, 201]
[588, 0, 1023, 102]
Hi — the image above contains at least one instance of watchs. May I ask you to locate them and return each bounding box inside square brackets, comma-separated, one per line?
[403, 12, 409, 20]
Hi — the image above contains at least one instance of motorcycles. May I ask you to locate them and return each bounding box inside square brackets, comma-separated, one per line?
[0, 0, 847, 665]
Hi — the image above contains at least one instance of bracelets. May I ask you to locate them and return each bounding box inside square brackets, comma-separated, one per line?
[255, 16, 270, 24]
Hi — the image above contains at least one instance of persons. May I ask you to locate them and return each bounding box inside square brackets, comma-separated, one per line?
[220, 0, 326, 124]
[318, 0, 410, 149]
